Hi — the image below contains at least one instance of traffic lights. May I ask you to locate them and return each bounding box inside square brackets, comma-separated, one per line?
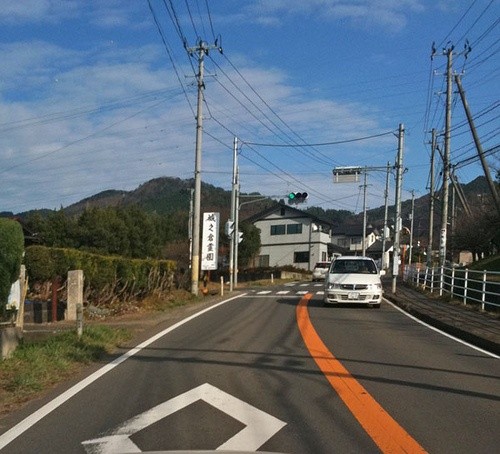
[289, 192, 308, 200]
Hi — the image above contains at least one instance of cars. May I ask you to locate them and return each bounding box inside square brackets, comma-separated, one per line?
[324, 256, 383, 308]
[312, 261, 331, 281]
